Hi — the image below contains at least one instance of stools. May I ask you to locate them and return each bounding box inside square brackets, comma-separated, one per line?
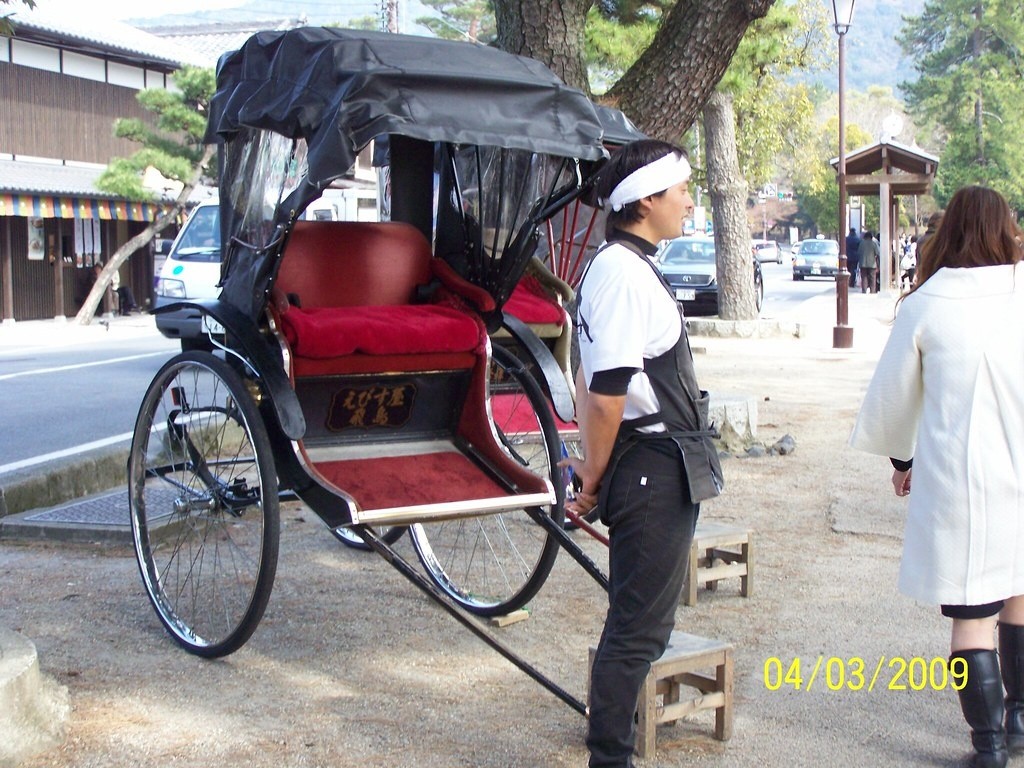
[589, 633, 736, 757]
[682, 520, 753, 607]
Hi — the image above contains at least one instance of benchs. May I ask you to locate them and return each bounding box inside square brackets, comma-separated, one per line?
[486, 227, 572, 337]
[273, 220, 494, 370]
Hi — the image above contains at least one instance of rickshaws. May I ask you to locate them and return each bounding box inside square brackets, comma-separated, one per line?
[126, 26, 612, 719]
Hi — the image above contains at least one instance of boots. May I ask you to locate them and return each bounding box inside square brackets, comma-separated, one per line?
[947, 649, 1008, 768]
[996, 620, 1024, 753]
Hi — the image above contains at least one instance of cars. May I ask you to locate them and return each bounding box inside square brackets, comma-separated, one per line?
[793, 240, 839, 281]
[655, 238, 763, 317]
[753, 240, 782, 264]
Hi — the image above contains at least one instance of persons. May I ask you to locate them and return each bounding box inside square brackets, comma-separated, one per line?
[915, 211, 945, 264]
[849, 185, 1024, 768]
[845, 228, 918, 293]
[557, 140, 722, 767]
[92, 262, 142, 316]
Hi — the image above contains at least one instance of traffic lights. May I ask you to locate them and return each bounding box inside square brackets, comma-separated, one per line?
[778, 192, 793, 201]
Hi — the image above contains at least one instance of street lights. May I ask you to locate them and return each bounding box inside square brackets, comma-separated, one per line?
[833, 0, 855, 348]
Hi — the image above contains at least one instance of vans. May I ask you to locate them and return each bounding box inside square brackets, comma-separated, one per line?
[155, 198, 338, 354]
